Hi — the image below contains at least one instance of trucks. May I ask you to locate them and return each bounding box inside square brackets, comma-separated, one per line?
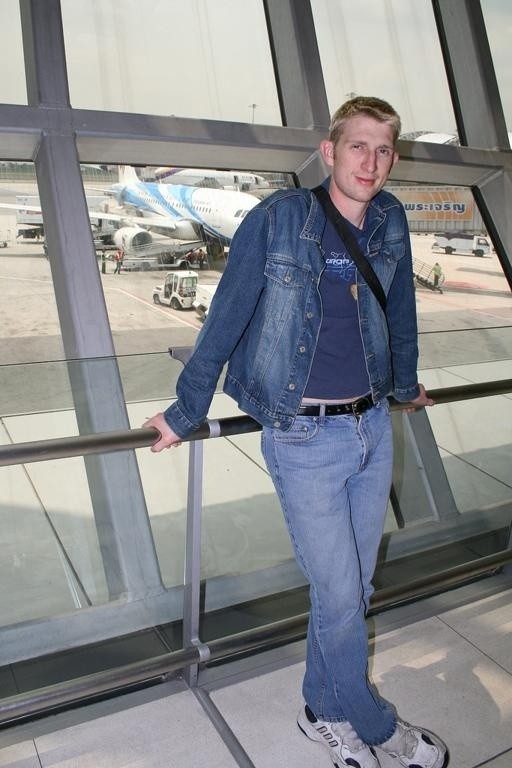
[120, 257, 201, 271]
[431, 234, 490, 256]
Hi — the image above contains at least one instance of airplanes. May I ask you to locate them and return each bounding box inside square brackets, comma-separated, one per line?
[0, 165, 270, 257]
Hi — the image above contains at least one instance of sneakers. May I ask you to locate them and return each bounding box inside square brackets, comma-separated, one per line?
[297, 703, 448, 768]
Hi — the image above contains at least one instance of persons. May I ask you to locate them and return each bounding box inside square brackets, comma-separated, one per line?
[142, 95, 448, 767]
[101, 249, 124, 274]
[431, 262, 442, 288]
[185, 249, 206, 269]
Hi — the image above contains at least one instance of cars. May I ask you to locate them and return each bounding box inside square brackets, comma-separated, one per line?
[153, 271, 198, 310]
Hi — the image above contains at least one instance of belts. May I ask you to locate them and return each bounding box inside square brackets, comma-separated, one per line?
[297, 393, 374, 416]
